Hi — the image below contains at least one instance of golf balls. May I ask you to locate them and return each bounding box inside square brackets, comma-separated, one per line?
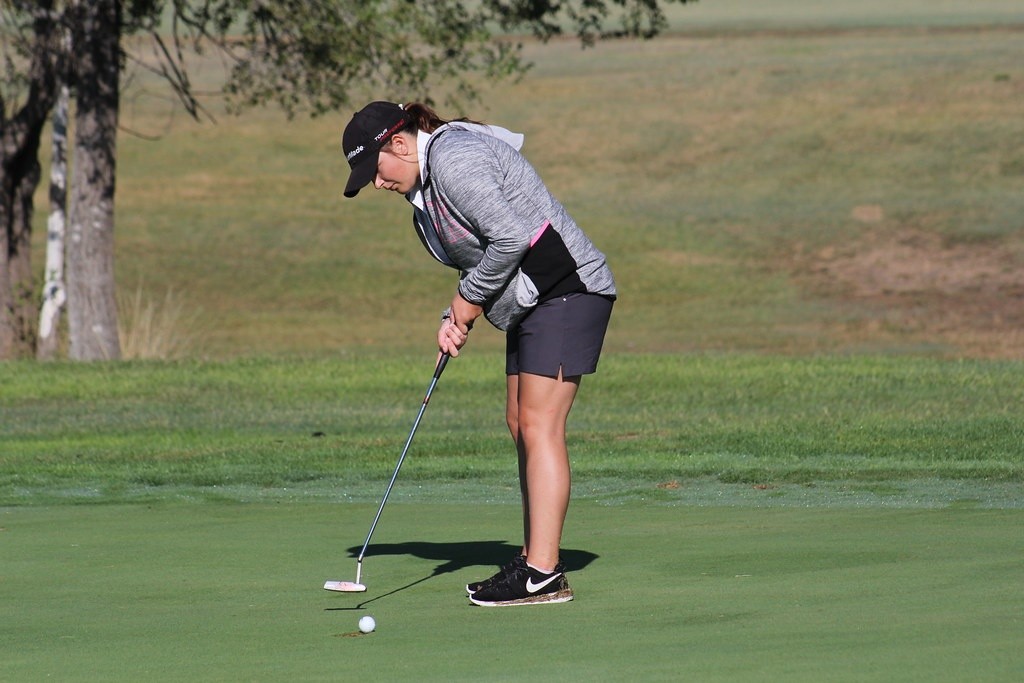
[358, 616, 376, 633]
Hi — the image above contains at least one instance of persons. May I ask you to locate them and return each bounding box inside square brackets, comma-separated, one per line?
[340, 99, 618, 607]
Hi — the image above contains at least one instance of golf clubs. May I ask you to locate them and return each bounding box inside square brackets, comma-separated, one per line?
[324, 349, 451, 592]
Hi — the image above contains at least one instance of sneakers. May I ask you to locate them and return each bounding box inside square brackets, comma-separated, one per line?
[466, 555, 573, 606]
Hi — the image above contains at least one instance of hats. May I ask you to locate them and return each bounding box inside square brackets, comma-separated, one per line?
[342, 101, 412, 199]
